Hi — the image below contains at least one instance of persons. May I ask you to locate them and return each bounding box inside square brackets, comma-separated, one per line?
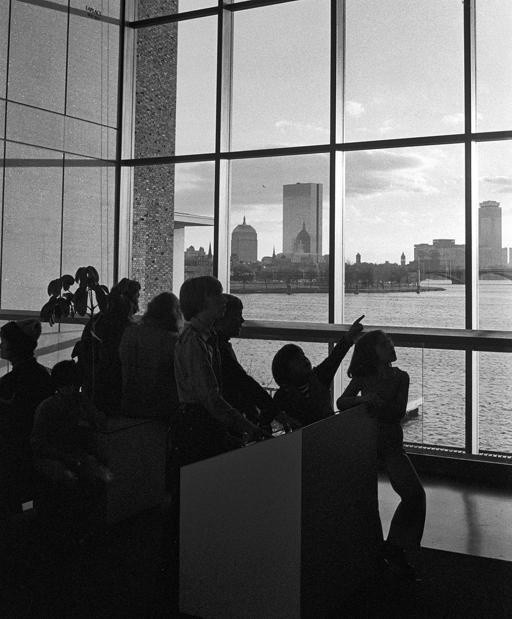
[176, 276, 264, 449]
[339, 328, 426, 584]
[273, 314, 365, 421]
[0, 320, 49, 475]
[221, 293, 283, 426]
[32, 361, 113, 513]
[80, 279, 142, 408]
[121, 292, 184, 418]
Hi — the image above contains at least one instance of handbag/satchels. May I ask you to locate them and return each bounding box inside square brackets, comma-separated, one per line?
[172, 402, 223, 463]
